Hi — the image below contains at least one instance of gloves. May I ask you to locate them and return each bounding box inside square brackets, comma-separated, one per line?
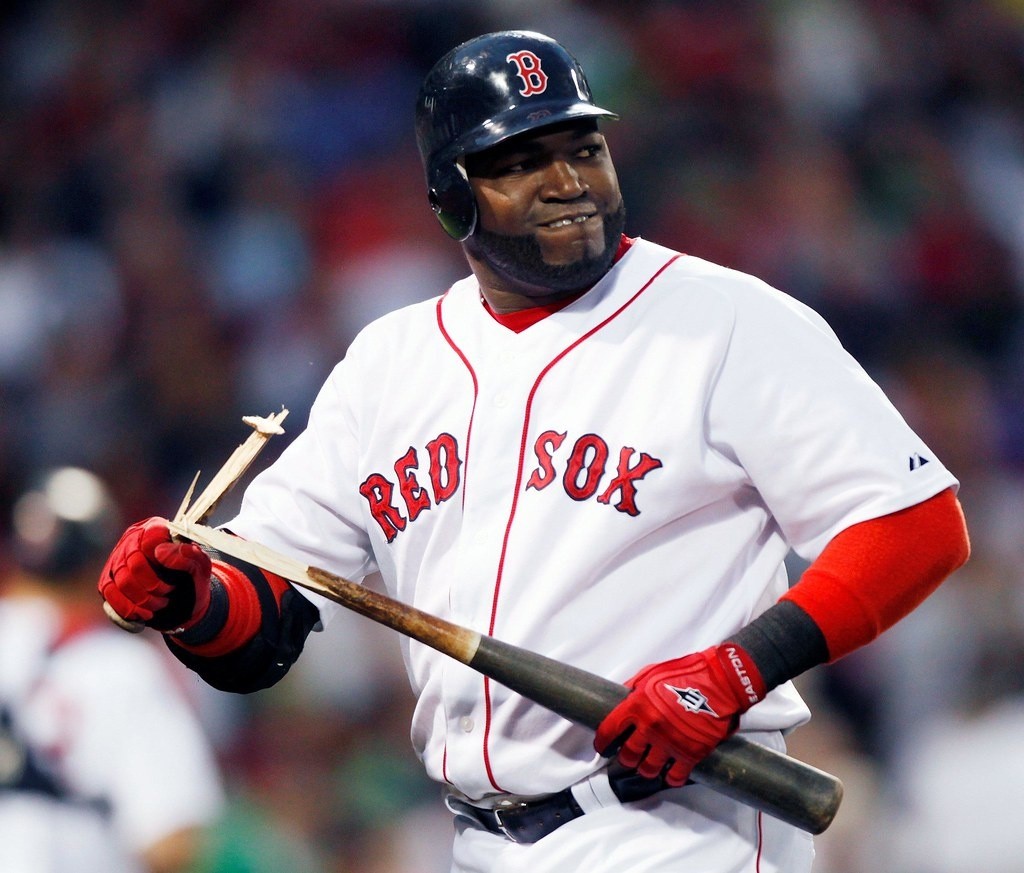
[592, 642, 763, 789]
[98, 516, 214, 629]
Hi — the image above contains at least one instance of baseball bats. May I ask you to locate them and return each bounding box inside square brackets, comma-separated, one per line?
[100, 407, 846, 837]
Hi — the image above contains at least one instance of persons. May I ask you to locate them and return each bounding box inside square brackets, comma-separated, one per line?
[95, 31, 973, 873]
[0, 466, 223, 872]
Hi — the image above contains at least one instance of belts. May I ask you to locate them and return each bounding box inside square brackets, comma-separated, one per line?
[453, 765, 671, 846]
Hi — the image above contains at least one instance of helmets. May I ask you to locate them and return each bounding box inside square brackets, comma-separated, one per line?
[416, 30, 620, 243]
